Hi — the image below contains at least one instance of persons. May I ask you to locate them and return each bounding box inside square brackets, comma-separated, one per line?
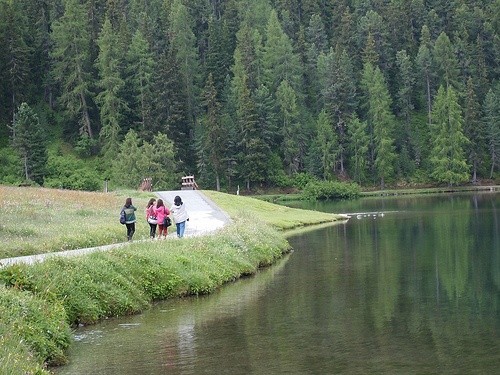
[120, 198, 137, 243]
[169, 196, 189, 239]
[155, 199, 170, 241]
[145, 198, 157, 239]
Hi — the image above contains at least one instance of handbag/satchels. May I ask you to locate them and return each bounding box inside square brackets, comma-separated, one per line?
[120, 208, 126, 224]
[162, 217, 171, 227]
[148, 216, 158, 224]
